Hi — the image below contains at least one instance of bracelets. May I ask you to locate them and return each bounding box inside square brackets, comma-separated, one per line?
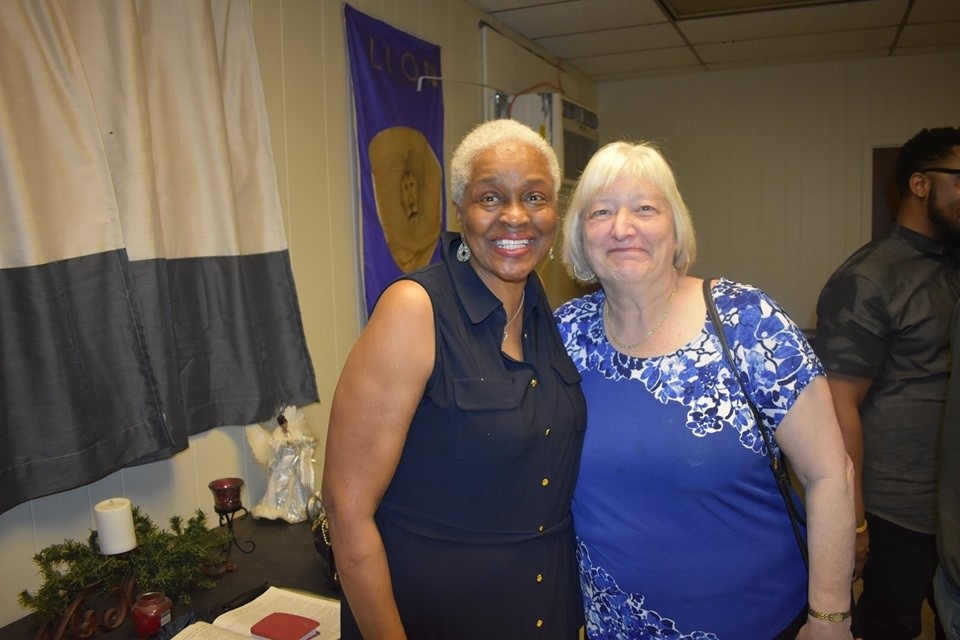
[855, 520, 868, 533]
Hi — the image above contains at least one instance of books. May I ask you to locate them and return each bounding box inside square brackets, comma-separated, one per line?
[170, 586, 341, 640]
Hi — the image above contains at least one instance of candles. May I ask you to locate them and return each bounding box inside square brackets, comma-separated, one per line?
[93, 498, 136, 555]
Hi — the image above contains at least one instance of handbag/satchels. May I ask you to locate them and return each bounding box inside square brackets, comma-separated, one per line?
[311, 513, 341, 591]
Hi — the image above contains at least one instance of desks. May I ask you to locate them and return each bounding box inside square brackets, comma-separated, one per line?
[0, 510, 361, 640]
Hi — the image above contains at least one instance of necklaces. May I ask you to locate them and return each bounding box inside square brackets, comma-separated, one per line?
[502, 290, 525, 342]
[607, 288, 674, 348]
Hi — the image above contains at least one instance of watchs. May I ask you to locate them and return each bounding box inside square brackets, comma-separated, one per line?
[807, 606, 852, 622]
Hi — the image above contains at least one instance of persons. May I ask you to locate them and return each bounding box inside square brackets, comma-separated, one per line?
[816, 127, 960, 640]
[323, 119, 588, 640]
[245, 404, 319, 523]
[552, 140, 855, 640]
